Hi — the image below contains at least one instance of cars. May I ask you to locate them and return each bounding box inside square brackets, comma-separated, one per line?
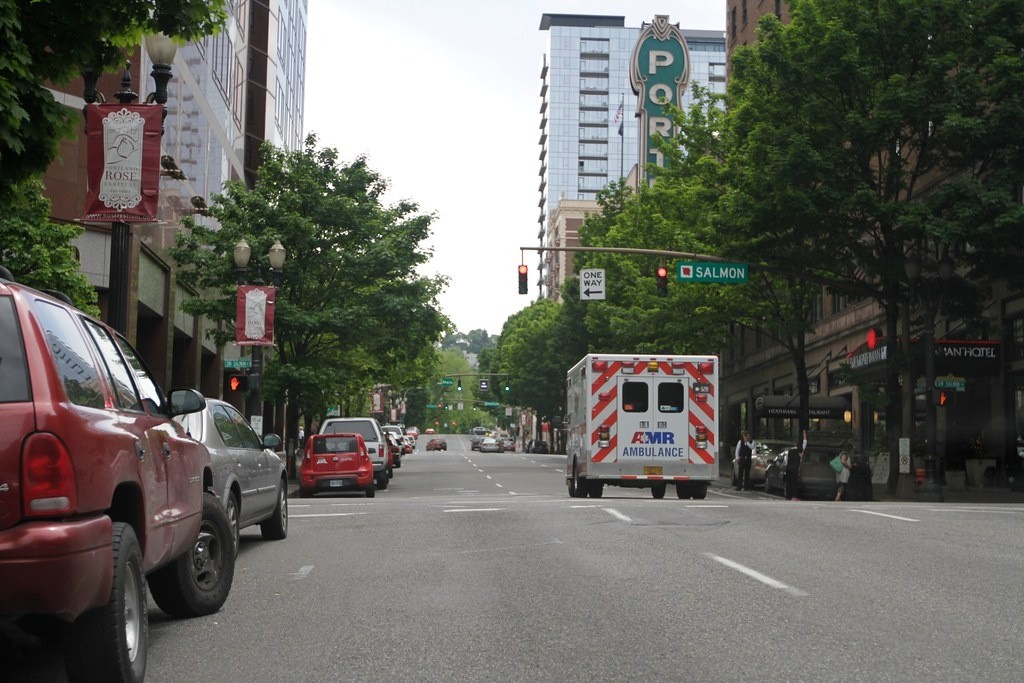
[731, 439, 797, 489]
[319, 417, 391, 490]
[764, 430, 855, 495]
[381, 426, 446, 478]
[166, 397, 289, 560]
[299, 433, 376, 498]
[470, 427, 548, 454]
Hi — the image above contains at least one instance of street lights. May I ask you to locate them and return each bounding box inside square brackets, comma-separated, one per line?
[235, 238, 286, 440]
[905, 252, 954, 502]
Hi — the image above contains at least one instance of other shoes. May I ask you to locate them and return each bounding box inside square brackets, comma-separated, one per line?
[745, 488, 750, 491]
[735, 487, 741, 491]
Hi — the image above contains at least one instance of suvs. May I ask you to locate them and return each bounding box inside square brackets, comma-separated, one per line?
[0, 264, 236, 683]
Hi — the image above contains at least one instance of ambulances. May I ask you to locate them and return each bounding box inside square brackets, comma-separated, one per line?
[550, 354, 719, 497]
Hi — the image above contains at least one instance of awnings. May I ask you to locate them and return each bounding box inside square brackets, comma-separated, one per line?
[755, 345, 850, 418]
[849, 300, 1001, 385]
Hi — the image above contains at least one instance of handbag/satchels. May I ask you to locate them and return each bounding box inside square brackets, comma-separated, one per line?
[830, 451, 849, 473]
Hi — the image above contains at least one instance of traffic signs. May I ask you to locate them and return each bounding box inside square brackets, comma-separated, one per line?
[579, 269, 606, 301]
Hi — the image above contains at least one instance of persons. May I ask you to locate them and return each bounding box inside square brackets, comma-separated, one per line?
[834, 443, 852, 501]
[783, 429, 807, 500]
[299, 426, 304, 450]
[735, 431, 754, 492]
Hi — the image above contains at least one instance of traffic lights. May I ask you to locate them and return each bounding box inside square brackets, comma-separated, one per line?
[936, 390, 952, 406]
[505, 381, 509, 391]
[230, 376, 248, 393]
[458, 380, 461, 391]
[519, 266, 527, 294]
[474, 405, 476, 410]
[445, 403, 448, 409]
[657, 268, 668, 296]
